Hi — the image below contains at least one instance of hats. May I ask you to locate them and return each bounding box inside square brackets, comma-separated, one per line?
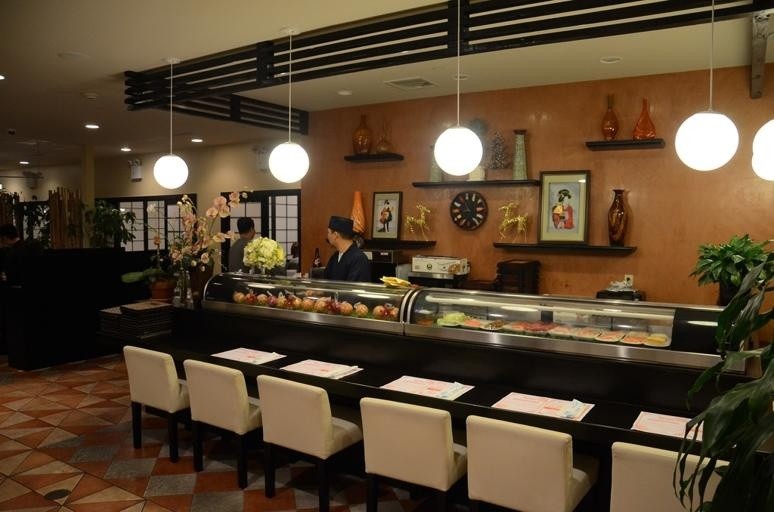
[328, 215, 353, 234]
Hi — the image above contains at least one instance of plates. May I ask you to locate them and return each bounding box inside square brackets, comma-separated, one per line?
[436, 318, 672, 348]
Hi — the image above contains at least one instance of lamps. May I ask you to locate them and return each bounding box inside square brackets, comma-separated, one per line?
[268, 28, 310, 183]
[153, 58, 189, 189]
[675, 0, 739, 171]
[435, 1, 483, 176]
[752, 119, 774, 183]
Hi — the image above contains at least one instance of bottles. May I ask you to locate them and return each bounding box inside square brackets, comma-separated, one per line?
[312, 247, 321, 268]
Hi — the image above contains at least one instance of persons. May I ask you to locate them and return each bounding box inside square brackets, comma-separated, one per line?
[326, 214, 379, 282]
[229, 217, 256, 273]
[1, 223, 59, 371]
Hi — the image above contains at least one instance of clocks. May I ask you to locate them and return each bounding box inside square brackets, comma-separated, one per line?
[450, 191, 488, 231]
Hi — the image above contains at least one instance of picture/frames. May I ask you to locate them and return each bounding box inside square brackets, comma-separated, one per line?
[537, 170, 591, 244]
[371, 191, 403, 240]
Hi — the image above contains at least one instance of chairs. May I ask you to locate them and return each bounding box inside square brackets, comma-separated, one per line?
[257, 374, 363, 512]
[360, 397, 466, 511]
[184, 359, 261, 489]
[609, 442, 730, 511]
[123, 345, 190, 462]
[466, 414, 604, 512]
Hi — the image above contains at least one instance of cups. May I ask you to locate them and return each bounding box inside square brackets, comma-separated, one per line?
[286, 269, 297, 278]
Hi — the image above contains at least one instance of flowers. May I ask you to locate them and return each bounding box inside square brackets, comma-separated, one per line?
[242, 236, 286, 269]
[169, 185, 255, 270]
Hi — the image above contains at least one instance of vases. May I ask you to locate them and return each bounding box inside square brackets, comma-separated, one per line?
[188, 256, 214, 292]
